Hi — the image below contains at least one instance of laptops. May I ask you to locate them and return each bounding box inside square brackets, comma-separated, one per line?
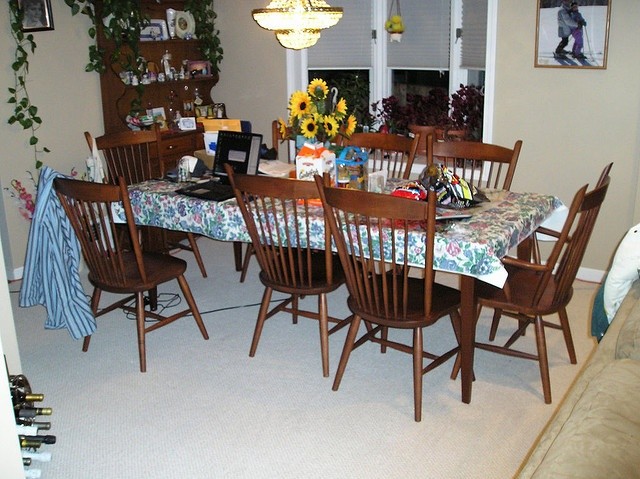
[173, 130, 263, 202]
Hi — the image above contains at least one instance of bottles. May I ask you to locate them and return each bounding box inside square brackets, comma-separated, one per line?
[24, 468, 41, 478]
[17, 435, 57, 444]
[15, 424, 38, 437]
[15, 419, 51, 431]
[19, 439, 42, 448]
[26, 407, 52, 416]
[22, 458, 32, 466]
[10, 388, 44, 406]
[14, 408, 38, 418]
[20, 450, 52, 462]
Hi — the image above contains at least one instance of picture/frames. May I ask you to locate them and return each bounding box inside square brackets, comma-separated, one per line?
[17, 0, 55, 32]
[534, 0, 611, 70]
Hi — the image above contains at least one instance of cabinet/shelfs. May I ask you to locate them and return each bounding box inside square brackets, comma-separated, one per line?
[105, 136, 195, 254]
[196, 131, 208, 151]
[94, 0, 226, 133]
[0, 243, 27, 479]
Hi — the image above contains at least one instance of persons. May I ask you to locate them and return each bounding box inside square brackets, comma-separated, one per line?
[553, 0, 583, 58]
[20, 0, 45, 28]
[569, 2, 586, 58]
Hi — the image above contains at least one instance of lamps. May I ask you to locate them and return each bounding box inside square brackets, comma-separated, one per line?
[252, 0, 343, 50]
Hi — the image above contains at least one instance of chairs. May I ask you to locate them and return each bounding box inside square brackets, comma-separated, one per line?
[225, 163, 375, 377]
[53, 178, 208, 373]
[313, 174, 475, 423]
[340, 126, 420, 178]
[240, 120, 346, 283]
[427, 137, 522, 189]
[450, 161, 612, 405]
[85, 124, 207, 279]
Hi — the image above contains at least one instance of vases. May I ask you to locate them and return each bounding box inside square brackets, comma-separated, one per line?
[435, 128, 468, 166]
[409, 124, 436, 155]
[384, 134, 405, 157]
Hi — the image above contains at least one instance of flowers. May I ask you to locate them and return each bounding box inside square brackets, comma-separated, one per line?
[277, 78, 354, 138]
[6, 168, 78, 223]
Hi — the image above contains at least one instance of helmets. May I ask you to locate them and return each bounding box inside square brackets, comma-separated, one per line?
[562, 0, 574, 11]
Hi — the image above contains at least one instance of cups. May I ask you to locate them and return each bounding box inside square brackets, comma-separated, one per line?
[175, 158, 190, 184]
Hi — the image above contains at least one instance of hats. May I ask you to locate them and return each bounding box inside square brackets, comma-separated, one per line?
[166, 155, 205, 183]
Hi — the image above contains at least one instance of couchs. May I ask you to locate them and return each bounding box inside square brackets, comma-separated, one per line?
[517, 224, 640, 479]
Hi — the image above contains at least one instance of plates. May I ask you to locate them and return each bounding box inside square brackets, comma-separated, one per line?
[175, 9, 196, 40]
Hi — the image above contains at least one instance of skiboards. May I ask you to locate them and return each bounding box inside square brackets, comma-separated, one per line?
[539, 55, 599, 61]
[540, 51, 601, 54]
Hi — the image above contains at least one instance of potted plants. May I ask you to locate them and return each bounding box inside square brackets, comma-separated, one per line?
[435, 83, 484, 164]
[371, 95, 416, 157]
[407, 91, 435, 156]
